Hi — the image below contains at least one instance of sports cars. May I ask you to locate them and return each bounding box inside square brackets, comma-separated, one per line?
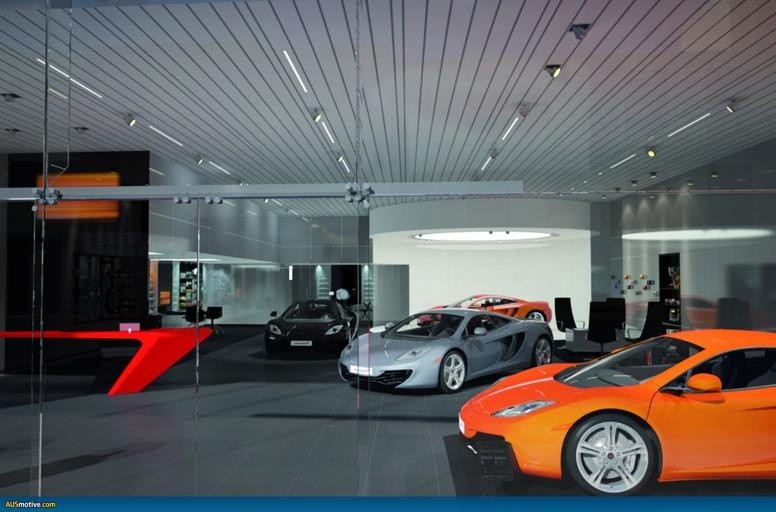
[265, 298, 350, 360]
[339, 295, 554, 393]
[458, 328, 776, 495]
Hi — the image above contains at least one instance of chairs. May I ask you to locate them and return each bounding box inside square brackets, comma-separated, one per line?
[606, 297, 626, 330]
[554, 297, 585, 349]
[718, 349, 747, 389]
[204, 306, 224, 335]
[185, 305, 205, 328]
[298, 303, 330, 317]
[431, 316, 481, 336]
[588, 301, 616, 356]
[626, 301, 670, 343]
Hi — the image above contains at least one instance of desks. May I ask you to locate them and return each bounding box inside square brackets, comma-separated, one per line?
[359, 309, 372, 321]
[0, 327, 214, 396]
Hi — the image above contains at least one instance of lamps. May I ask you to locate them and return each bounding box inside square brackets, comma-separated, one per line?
[570, 24, 589, 39]
[648, 148, 656, 158]
[725, 100, 738, 113]
[310, 111, 321, 121]
[125, 116, 136, 126]
[545, 63, 561, 78]
